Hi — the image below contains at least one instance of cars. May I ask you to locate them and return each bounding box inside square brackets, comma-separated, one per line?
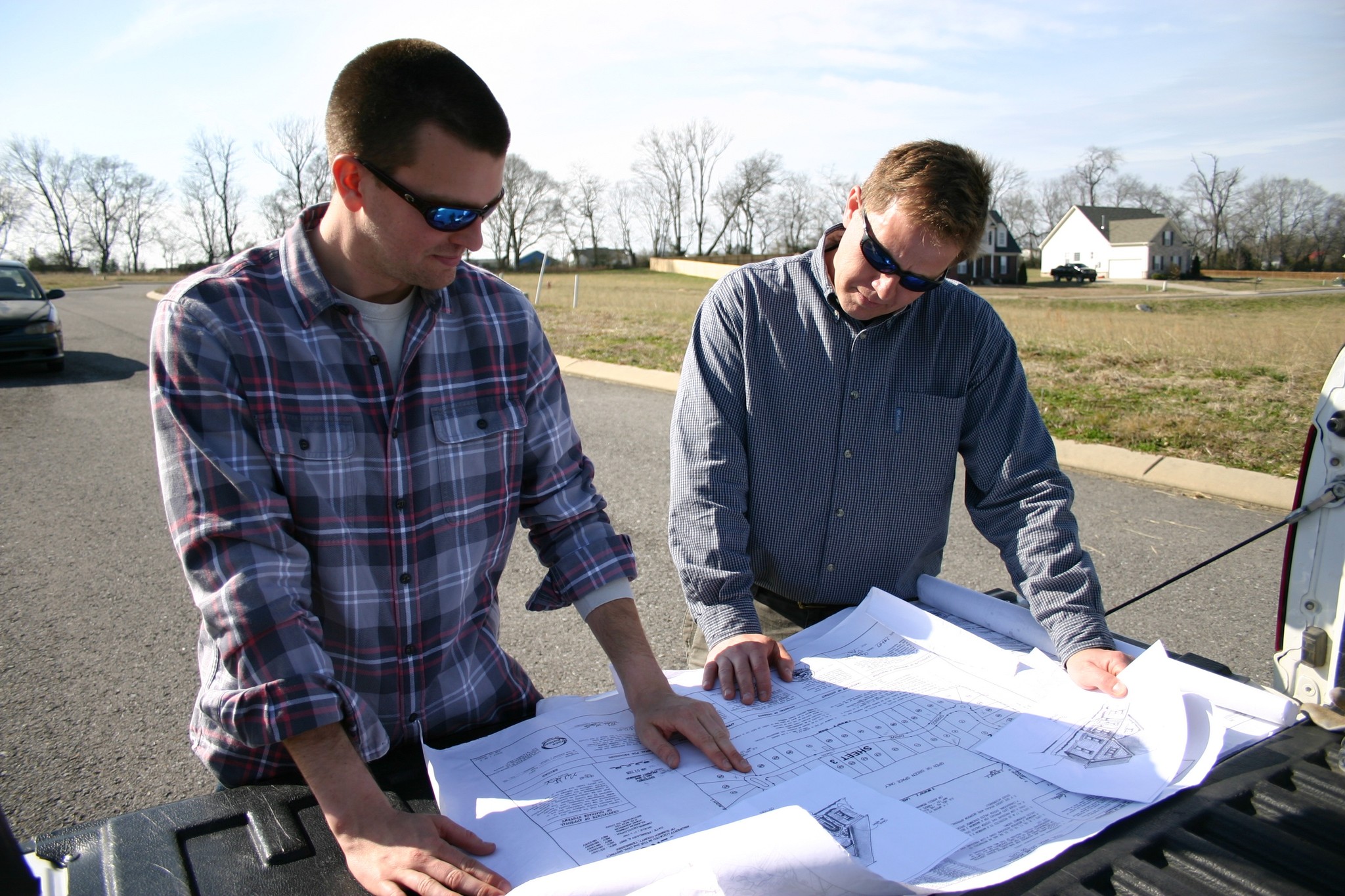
[0, 259, 67, 379]
[1050, 262, 1097, 283]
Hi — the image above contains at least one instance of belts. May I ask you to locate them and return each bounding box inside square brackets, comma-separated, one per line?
[759, 587, 859, 610]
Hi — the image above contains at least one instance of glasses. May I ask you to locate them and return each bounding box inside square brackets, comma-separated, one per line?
[353, 150, 506, 232]
[861, 206, 948, 292]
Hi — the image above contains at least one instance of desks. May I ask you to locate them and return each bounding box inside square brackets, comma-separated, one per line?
[0, 582, 1345, 895]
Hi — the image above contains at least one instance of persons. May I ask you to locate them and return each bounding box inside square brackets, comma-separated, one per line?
[147, 37, 751, 895]
[668, 135, 1140, 709]
